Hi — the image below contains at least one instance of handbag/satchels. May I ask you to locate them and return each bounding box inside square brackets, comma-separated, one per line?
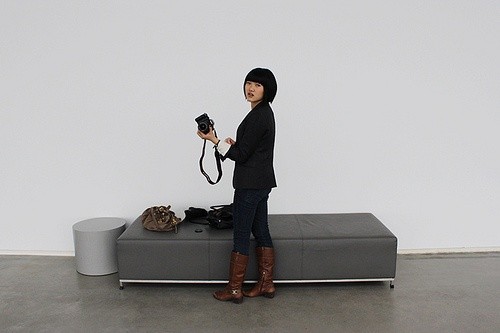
[208, 203, 234, 230]
[183, 207, 210, 225]
[141, 205, 181, 232]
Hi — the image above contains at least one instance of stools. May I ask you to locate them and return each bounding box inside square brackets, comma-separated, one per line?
[72, 217, 128, 276]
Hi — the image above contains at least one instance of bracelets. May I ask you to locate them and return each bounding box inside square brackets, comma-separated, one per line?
[213, 140, 221, 148]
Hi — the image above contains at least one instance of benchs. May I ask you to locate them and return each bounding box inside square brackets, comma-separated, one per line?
[116, 213, 398, 290]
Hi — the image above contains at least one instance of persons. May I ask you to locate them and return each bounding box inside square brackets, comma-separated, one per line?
[196, 68, 278, 304]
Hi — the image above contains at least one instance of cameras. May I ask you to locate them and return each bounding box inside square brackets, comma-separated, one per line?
[195, 113, 214, 135]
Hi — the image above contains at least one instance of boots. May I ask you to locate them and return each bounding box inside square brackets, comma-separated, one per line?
[213, 252, 249, 304]
[243, 247, 275, 298]
